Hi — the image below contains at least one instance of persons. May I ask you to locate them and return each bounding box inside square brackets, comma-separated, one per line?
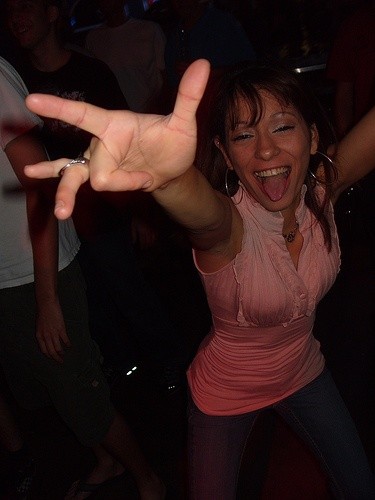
[0, 52, 170, 500]
[22, 58, 375, 500]
[2, 2, 157, 400]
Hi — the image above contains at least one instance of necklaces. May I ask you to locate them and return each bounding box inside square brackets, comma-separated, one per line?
[277, 223, 301, 243]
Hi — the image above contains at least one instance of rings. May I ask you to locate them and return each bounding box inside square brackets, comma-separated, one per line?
[57, 149, 91, 176]
